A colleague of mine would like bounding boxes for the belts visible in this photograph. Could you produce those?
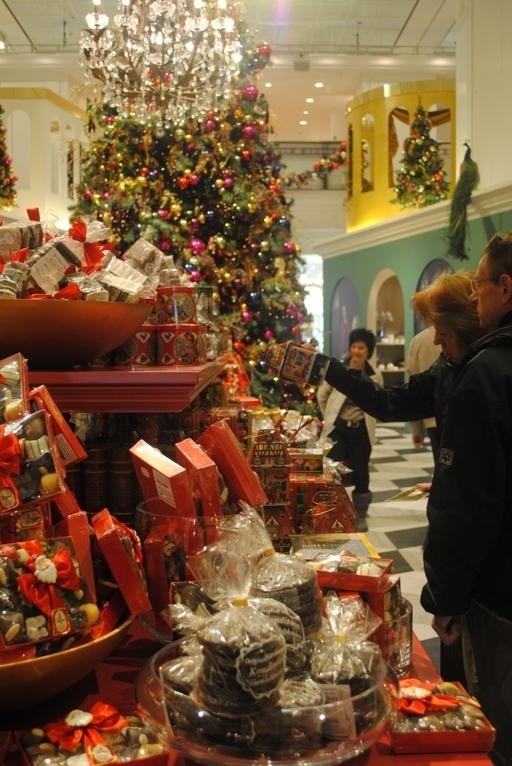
[335,416,366,430]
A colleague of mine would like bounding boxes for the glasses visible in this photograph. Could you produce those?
[469,275,499,294]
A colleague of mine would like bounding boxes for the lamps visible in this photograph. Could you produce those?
[74,0,245,135]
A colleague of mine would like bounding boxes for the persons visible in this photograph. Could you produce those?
[303,268,488,678]
[316,327,385,532]
[406,325,443,466]
[418,230,512,766]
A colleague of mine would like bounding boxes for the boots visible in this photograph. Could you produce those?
[352,488,371,532]
